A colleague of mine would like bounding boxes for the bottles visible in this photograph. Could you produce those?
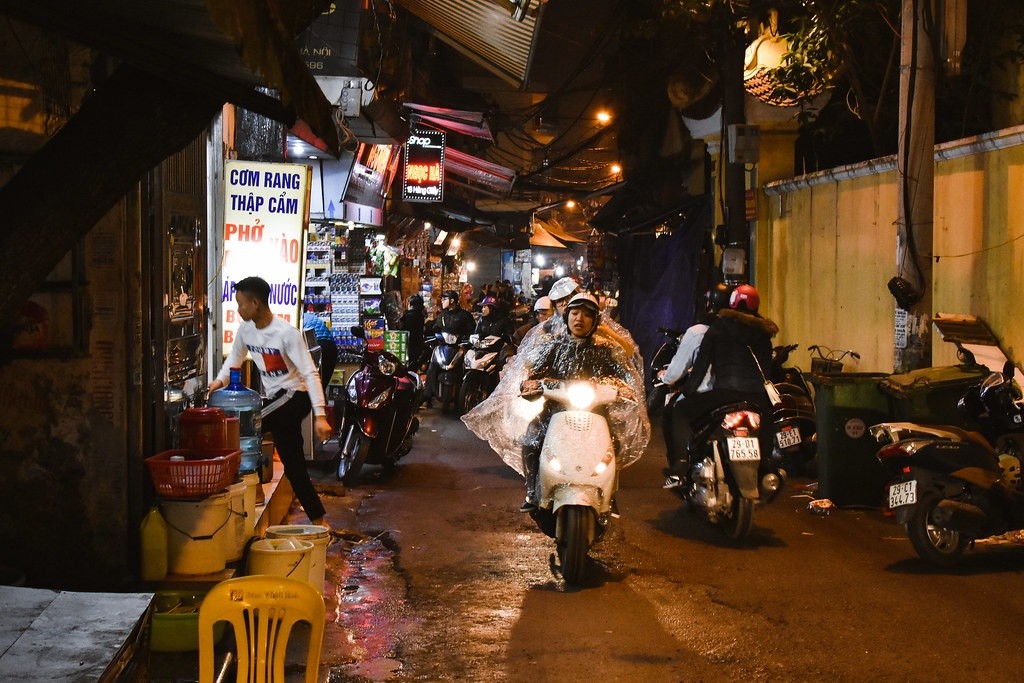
[322,244,366,273]
[140,507,168,579]
[333,327,365,363]
[208,366,261,483]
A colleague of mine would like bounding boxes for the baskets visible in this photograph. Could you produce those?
[811,358,842,375]
[145,447,243,498]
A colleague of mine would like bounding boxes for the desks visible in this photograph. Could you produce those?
[0,585,156,683]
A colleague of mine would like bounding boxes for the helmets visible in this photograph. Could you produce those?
[439,290,458,302]
[534,296,554,311]
[704,282,732,311]
[728,284,759,312]
[549,277,581,308]
[477,296,499,309]
[567,293,599,319]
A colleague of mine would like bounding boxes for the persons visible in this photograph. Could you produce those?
[398,276,558,402]
[663,285,784,491]
[499,277,644,519]
[657,282,735,477]
[205,276,334,532]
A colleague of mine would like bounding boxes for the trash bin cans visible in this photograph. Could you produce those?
[802,371,891,511]
[878,364,991,432]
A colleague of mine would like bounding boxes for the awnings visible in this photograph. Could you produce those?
[395,0,548,90]
[402,100,500,147]
[444,146,518,201]
[0,0,344,157]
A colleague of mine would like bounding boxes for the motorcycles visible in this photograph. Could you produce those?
[335,325,424,481]
[867,345,1024,564]
[422,330,517,410]
[651,326,820,543]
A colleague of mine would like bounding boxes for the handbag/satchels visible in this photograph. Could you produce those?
[764,380,782,406]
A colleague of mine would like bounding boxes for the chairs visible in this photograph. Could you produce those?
[199,574,326,683]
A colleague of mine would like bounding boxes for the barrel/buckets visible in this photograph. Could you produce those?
[226,478,248,563]
[156,489,232,575]
[265,525,331,597]
[247,539,315,621]
[239,471,260,539]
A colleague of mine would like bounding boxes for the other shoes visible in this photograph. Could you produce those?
[611,501,620,519]
[520,499,535,512]
[663,476,683,488]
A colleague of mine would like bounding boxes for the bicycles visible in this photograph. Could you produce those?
[806,344,861,379]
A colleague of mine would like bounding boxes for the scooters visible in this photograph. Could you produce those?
[517,379,633,587]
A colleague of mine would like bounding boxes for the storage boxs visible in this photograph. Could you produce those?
[381,329,408,363]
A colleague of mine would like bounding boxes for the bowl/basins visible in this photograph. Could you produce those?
[150,590,225,652]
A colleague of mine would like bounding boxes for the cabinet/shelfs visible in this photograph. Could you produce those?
[305,244,333,326]
